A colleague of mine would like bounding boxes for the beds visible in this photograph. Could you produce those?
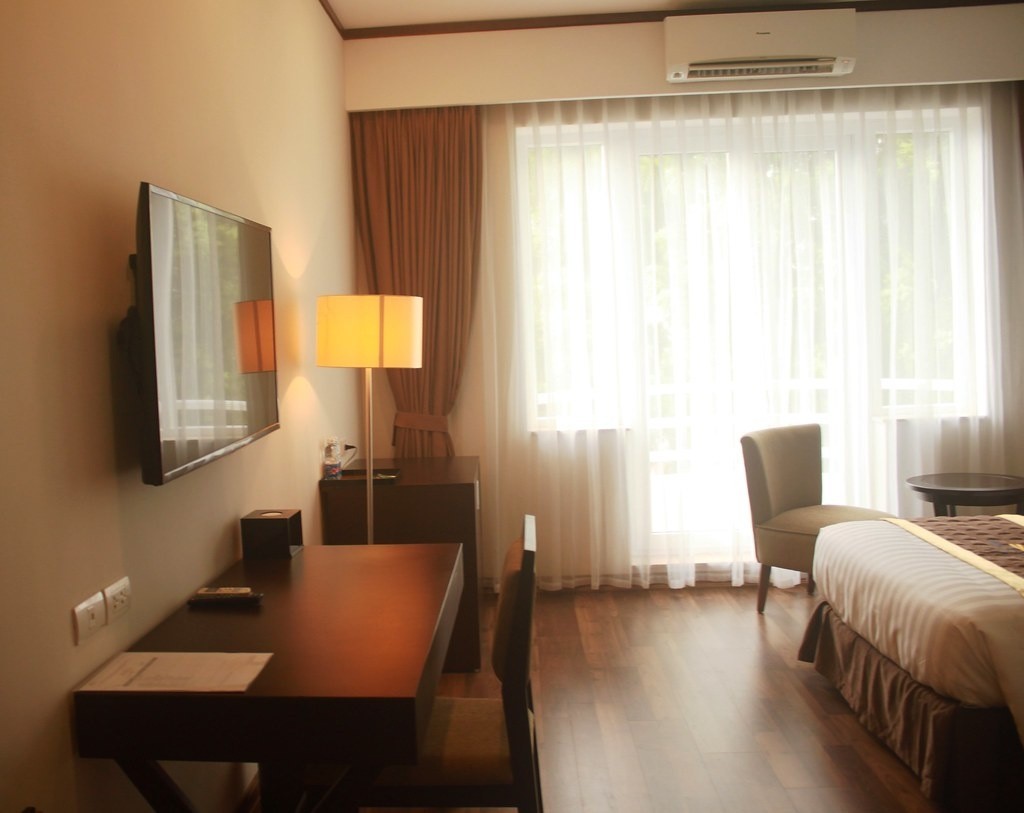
[796,514,1024,803]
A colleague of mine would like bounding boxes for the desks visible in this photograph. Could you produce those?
[72,542,464,813]
[319,455,482,674]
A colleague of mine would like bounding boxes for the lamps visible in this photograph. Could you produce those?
[314,295,422,546]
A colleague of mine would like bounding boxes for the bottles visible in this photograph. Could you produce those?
[325,436,341,480]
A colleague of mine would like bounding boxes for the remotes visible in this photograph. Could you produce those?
[186,586,264,609]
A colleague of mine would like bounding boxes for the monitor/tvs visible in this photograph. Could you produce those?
[116,181,280,487]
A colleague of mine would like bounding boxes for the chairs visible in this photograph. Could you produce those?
[333,514,544,813]
[739,423,899,613]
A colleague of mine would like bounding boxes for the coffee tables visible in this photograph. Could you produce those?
[905,472,1024,517]
